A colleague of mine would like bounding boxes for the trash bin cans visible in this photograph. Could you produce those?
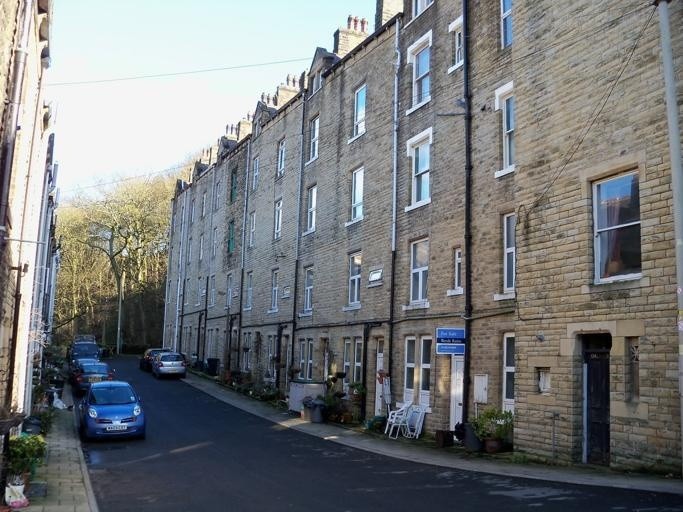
[21,417,41,435]
[464,422,481,451]
[206,358,219,375]
[312,401,324,422]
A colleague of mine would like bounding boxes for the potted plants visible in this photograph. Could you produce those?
[470,406,514,453]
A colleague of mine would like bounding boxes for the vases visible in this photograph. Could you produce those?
[8,473,28,495]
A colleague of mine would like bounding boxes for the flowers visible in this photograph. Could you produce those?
[3,432,48,472]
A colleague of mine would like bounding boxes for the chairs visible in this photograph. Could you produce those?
[382,397,429,440]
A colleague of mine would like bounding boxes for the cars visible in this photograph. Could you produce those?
[70,334,186,443]
